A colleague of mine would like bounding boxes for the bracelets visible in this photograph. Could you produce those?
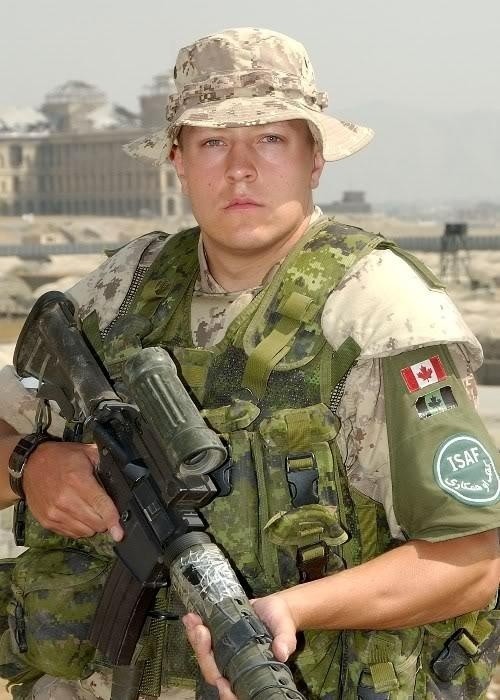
[9,429,62,500]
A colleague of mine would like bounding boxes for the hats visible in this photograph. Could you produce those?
[123,28,376,167]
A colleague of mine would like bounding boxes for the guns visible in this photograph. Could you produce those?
[13,290,306,700]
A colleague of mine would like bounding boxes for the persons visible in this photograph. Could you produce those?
[0,25,500,700]
